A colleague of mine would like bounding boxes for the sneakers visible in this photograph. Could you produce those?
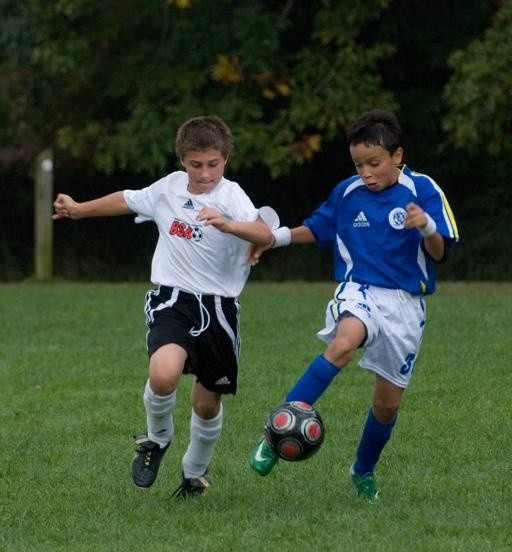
[349,460,379,503]
[178,471,216,500]
[248,434,281,476]
[129,434,171,489]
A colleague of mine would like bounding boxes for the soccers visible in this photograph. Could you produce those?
[265,401,324,462]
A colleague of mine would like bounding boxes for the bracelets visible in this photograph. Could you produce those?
[416,209,438,237]
[270,224,292,250]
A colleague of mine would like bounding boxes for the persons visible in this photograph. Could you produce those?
[51,115,281,502]
[244,107,461,500]
